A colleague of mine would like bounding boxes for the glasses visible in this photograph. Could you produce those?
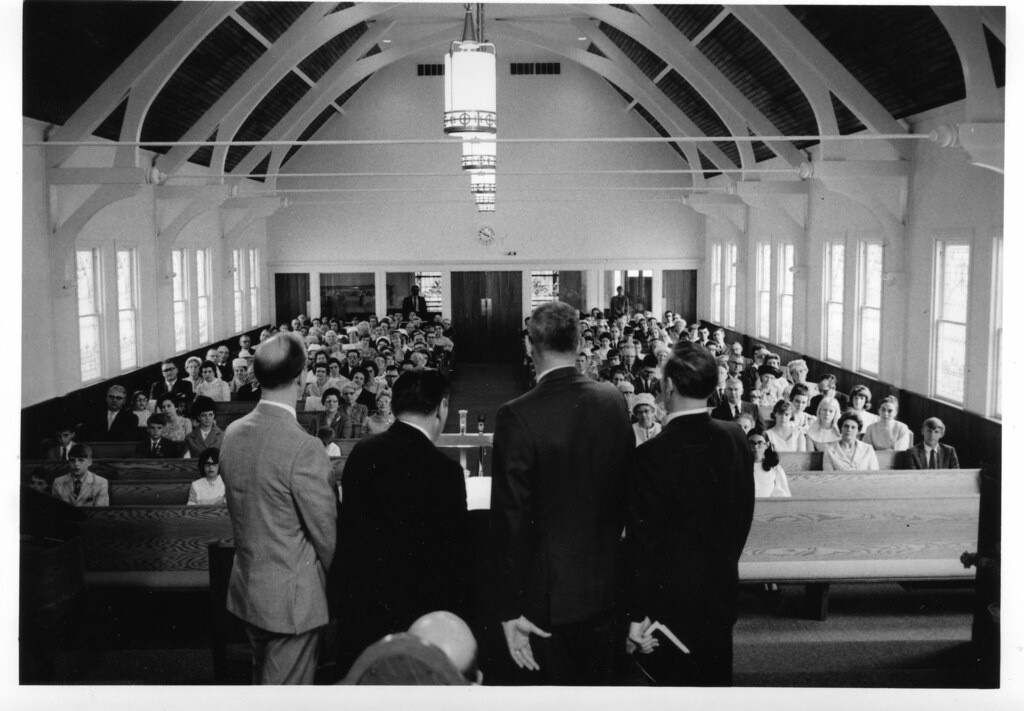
[204,462,218,467]
[751,441,766,448]
[325,399,339,404]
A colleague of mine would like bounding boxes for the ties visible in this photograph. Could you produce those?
[614,342,616,349]
[734,406,739,415]
[928,450,935,469]
[719,346,722,355]
[413,297,416,311]
[719,388,724,401]
[645,380,649,393]
[73,480,81,499]
[62,448,67,461]
[151,442,156,452]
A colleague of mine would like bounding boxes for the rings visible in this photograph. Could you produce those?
[515,650,521,653]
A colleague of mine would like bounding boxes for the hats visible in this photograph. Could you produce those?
[374,336,392,345]
[630,393,657,417]
[398,329,409,339]
[346,327,358,333]
[757,365,783,379]
[617,381,634,393]
[232,358,248,367]
[307,344,322,352]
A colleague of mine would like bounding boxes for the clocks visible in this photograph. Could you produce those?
[477,225,496,245]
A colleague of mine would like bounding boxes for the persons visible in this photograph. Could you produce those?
[220,303,755,686]
[521,286,960,497]
[29,284,455,507]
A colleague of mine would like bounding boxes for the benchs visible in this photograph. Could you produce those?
[20,398,321,600]
[648,451,982,622]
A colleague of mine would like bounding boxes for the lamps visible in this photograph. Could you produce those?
[443,3,497,214]
